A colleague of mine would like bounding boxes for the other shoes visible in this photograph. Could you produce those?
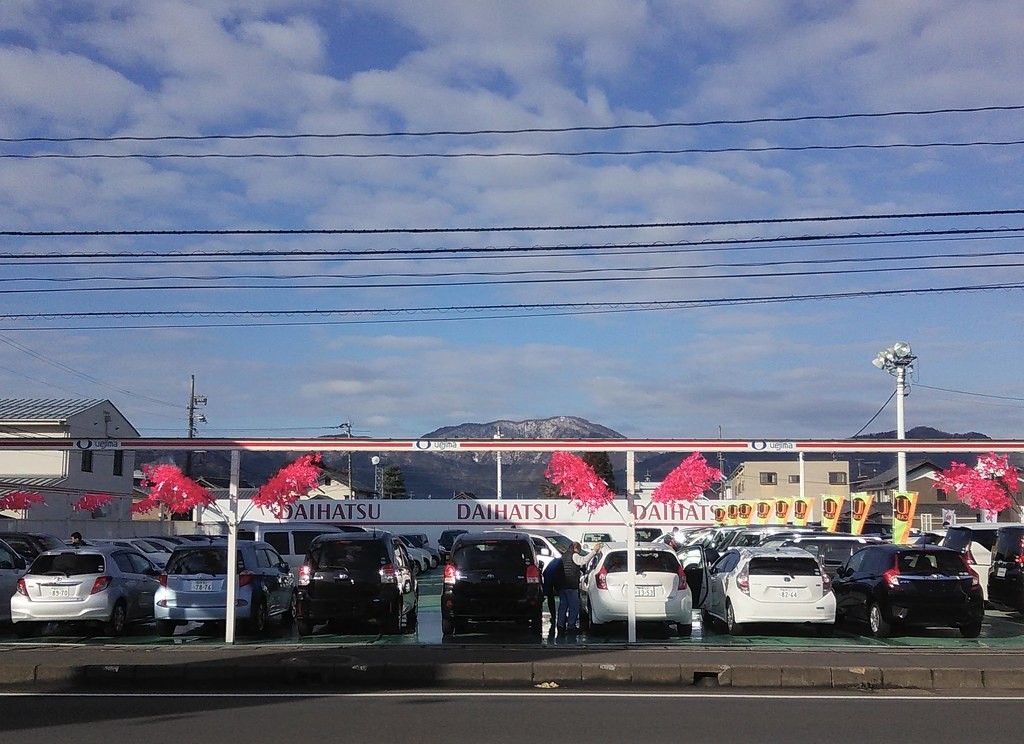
[567,627,580,630]
[558,625,566,630]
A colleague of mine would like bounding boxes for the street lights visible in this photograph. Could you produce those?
[874,341,919,502]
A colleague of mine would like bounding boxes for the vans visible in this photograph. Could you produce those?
[194,522,369,595]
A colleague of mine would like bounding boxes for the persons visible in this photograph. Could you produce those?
[942,521,949,529]
[70,532,86,546]
[664,527,682,552]
[543,541,600,635]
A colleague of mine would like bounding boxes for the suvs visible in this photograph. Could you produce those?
[438,532,550,640]
[295,530,421,634]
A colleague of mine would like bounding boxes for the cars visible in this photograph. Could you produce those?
[10,545,164,636]
[0,531,446,619]
[496,520,1024,620]
[703,547,839,635]
[579,543,710,637]
[154,538,298,638]
[832,543,985,639]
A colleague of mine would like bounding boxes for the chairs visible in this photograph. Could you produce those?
[641,558,662,570]
[611,557,626,568]
[601,536,605,540]
[587,537,592,542]
[464,548,481,567]
[915,557,937,571]
